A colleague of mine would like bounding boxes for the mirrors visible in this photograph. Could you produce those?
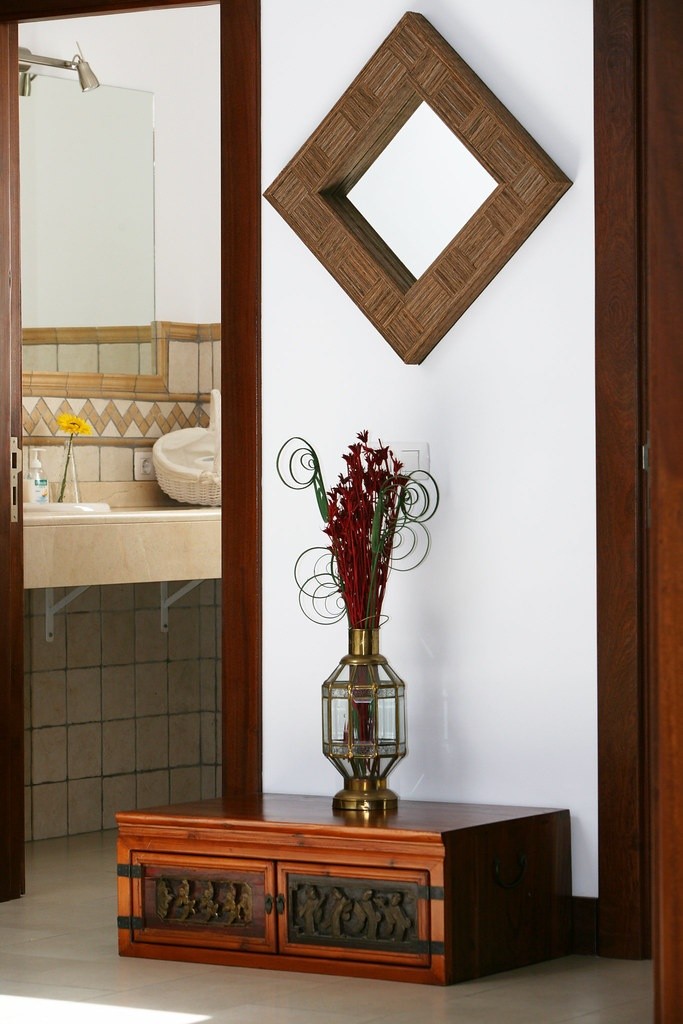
[18,76,158,378]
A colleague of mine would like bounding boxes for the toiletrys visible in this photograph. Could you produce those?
[29,447,50,503]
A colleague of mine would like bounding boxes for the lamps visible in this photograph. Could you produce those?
[18,47,100,97]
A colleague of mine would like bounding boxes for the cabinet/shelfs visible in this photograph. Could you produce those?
[115,794,571,986]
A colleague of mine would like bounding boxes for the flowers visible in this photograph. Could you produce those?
[58,411,91,503]
[276,430,439,774]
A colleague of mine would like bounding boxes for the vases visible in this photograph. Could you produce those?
[322,629,408,810]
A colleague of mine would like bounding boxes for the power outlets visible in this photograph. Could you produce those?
[133,452,157,481]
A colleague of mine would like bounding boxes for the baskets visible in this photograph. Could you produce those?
[152,388,222,507]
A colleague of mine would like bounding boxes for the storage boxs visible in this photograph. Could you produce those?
[153,427,222,506]
